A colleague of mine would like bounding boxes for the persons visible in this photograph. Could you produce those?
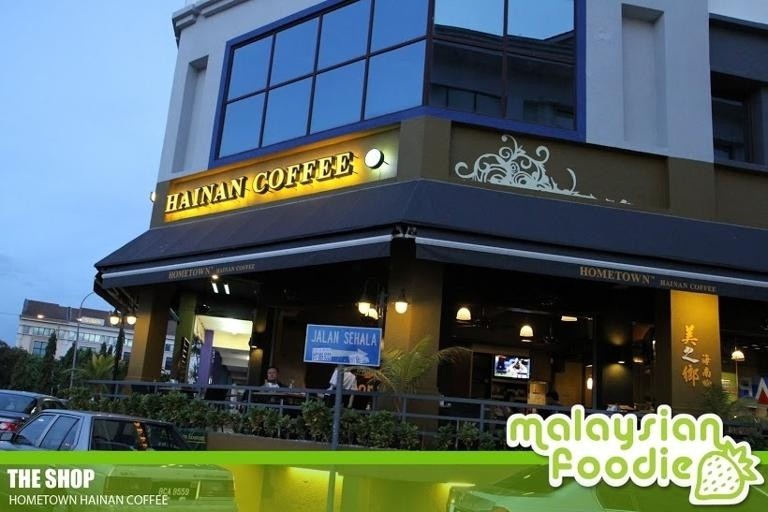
[325,367,358,409]
[504,390,518,413]
[536,390,563,420]
[267,368,296,387]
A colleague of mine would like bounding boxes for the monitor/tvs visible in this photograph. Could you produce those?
[492,353,532,382]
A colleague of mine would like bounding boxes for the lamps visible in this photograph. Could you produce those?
[586,374,594,391]
[109,296,136,325]
[355,288,409,320]
[730,346,745,396]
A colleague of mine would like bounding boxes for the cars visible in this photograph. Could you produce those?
[0,388,237,512]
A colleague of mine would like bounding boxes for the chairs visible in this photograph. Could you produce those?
[128,380,376,432]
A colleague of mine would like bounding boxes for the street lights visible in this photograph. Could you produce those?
[109,308,137,402]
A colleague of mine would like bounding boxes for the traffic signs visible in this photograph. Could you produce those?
[300,323,382,368]
